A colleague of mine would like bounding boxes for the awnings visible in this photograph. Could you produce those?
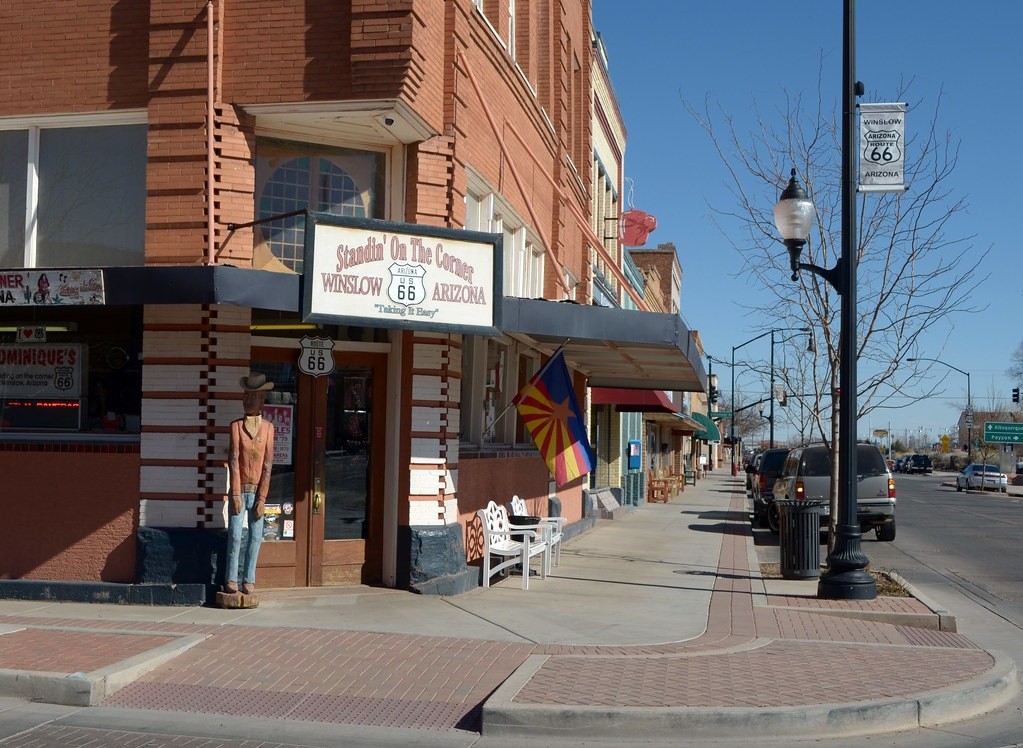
[691,412,721,441]
[592,387,678,413]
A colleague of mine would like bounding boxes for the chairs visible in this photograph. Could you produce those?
[682,463,698,487]
[479,501,550,590]
[647,465,686,504]
[506,495,567,576]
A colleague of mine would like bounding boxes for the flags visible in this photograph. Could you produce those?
[511,345,597,489]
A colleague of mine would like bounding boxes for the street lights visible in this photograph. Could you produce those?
[906,357,974,466]
[770,0,880,600]
[731,326,812,477]
[755,328,817,448]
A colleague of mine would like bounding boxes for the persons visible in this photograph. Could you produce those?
[223,371,275,593]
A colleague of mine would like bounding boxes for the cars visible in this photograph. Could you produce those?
[886,453,934,474]
[955,464,1007,493]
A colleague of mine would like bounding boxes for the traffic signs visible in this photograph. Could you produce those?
[984,421,1023,445]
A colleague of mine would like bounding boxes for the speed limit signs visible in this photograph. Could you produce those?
[855,100,909,194]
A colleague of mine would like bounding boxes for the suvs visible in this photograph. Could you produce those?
[745,448,791,529]
[766,438,898,541]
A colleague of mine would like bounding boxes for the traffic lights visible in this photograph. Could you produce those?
[710,385,719,404]
[963,444,967,450]
[1012,387,1020,403]
[835,386,840,393]
[779,390,788,406]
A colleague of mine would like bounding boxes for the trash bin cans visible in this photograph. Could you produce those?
[773,499,823,580]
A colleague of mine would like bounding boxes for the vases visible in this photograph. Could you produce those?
[507,515,540,577]
